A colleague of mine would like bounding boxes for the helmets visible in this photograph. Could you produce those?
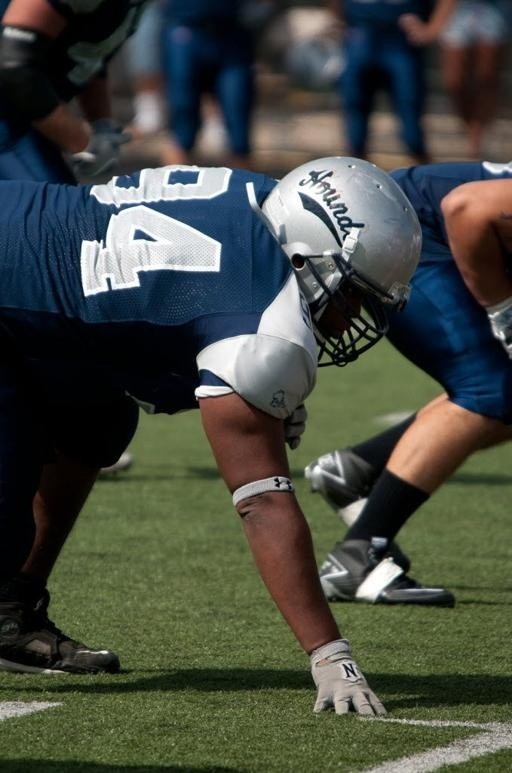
[262,156,422,320]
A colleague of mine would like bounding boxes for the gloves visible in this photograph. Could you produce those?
[283,404,306,449]
[310,639,388,714]
[74,118,130,180]
[488,304,512,362]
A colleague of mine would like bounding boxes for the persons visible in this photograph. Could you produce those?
[305,1,454,166]
[149,2,294,172]
[298,157,510,606]
[1,0,149,683]
[2,153,426,722]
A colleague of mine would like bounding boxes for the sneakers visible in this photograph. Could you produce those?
[2,595,118,676]
[303,445,411,573]
[101,452,130,477]
[318,537,455,606]
